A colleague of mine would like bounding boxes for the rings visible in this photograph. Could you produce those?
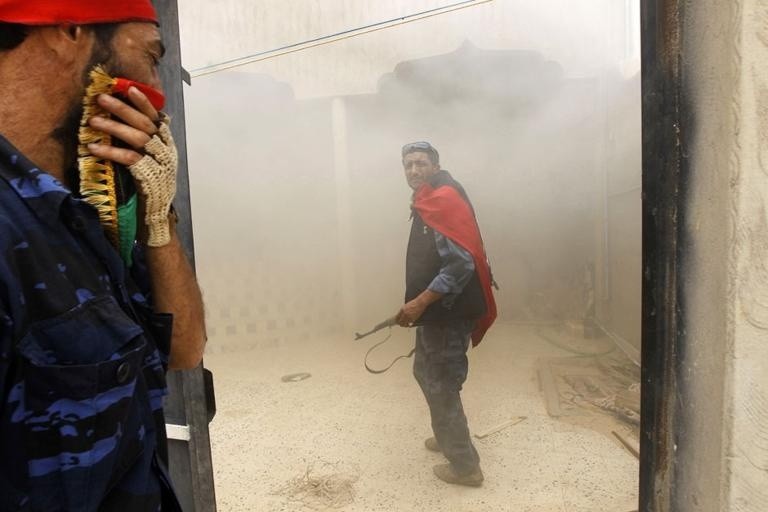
[407,322,414,326]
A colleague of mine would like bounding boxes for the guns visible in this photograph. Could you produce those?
[354,313,398,340]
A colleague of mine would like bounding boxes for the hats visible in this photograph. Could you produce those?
[1,1,160,27]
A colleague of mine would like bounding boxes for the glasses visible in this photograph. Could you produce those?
[404,141,430,151]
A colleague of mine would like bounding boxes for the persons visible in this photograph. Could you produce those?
[0,0,211,511]
[391,141,496,487]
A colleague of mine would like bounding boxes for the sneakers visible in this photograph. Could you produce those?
[426,437,440,452]
[434,464,484,486]
